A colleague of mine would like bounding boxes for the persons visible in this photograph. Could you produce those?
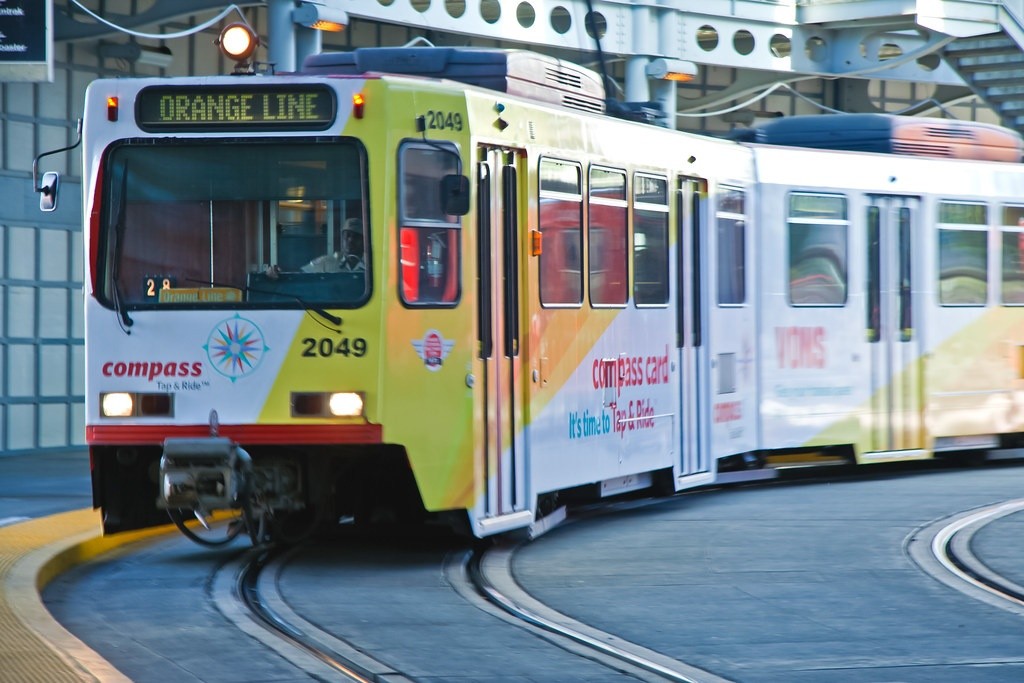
[299,218,366,271]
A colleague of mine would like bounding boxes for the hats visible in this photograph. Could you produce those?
[341,218,364,235]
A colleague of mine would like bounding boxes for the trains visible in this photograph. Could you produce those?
[30,24,1024,544]
